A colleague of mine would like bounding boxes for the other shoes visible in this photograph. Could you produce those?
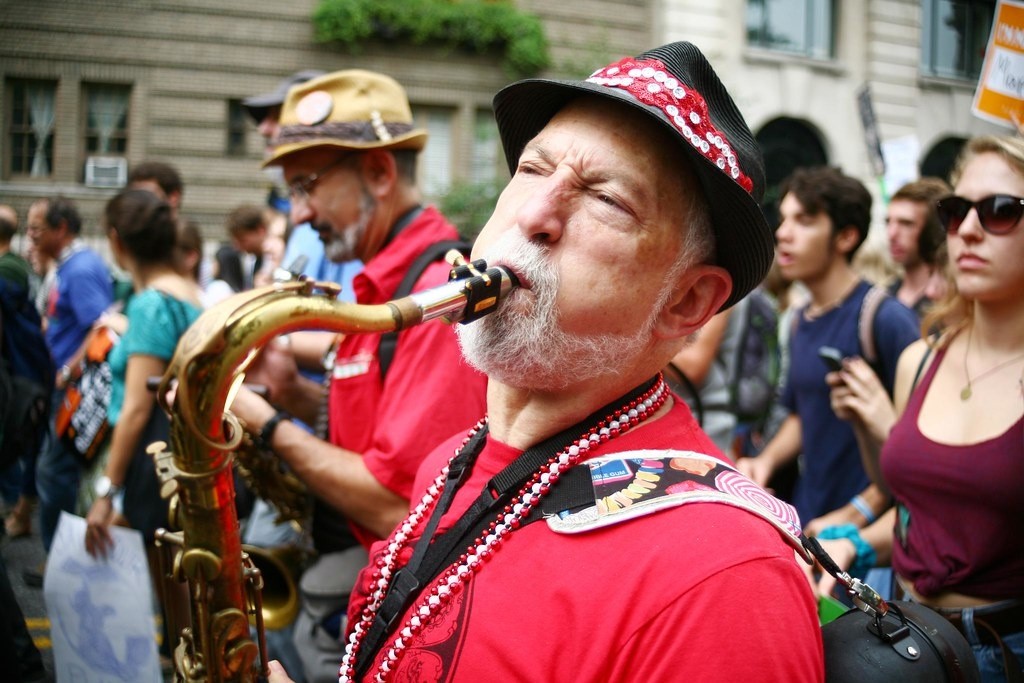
[6,499,34,537]
[22,562,46,587]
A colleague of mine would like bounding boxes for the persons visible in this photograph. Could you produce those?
[0,64,489,683]
[662,114,1024,683]
[332,41,825,683]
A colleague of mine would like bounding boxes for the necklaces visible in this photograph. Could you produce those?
[961,328,1024,399]
[337,372,669,683]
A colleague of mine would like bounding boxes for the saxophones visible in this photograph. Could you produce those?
[149,377,318,632]
[145,249,522,683]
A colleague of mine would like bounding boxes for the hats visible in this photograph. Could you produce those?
[260,68,426,172]
[494,42,777,304]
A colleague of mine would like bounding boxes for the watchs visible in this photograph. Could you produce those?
[61,365,73,382]
[93,475,118,499]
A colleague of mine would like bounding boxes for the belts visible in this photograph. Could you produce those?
[947,603,1024,683]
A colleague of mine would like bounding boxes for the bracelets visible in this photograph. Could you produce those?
[281,335,290,348]
[850,496,876,523]
[258,410,289,443]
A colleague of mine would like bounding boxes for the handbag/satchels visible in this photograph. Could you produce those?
[823,602,983,683]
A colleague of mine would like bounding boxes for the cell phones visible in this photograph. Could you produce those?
[817,346,846,387]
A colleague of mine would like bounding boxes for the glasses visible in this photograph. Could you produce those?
[937,193,1024,236]
[277,152,352,200]
